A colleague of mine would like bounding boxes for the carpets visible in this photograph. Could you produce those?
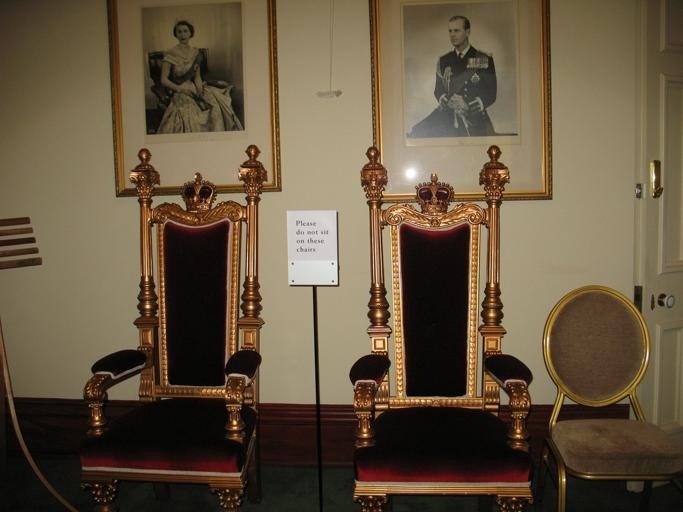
[457,52,462,61]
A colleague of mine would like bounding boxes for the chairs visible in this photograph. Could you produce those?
[80,145,268,509]
[350,144,536,508]
[534,286,683,510]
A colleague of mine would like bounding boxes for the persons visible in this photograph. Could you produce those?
[410,13,498,137]
[160,17,226,133]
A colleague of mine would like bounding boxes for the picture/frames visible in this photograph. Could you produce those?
[108,0,282,197]
[369,2,553,201]
